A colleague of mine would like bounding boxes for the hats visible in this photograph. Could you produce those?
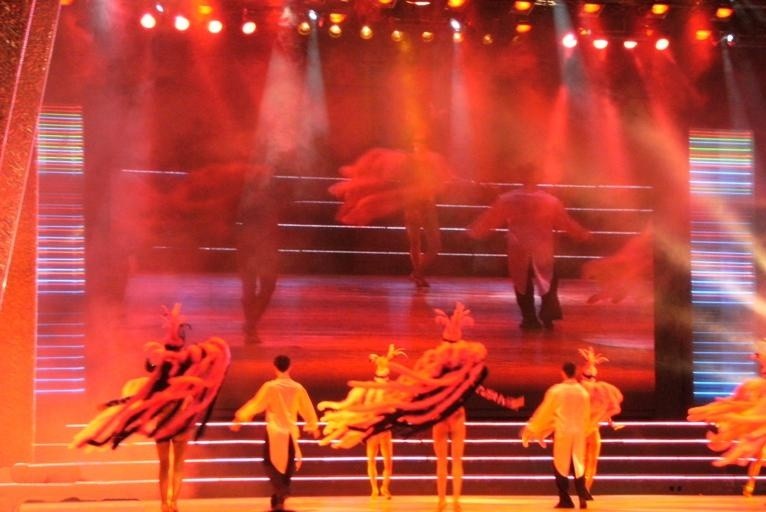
[164,336,183,346]
[583,365,596,377]
[374,368,390,376]
[442,326,462,340]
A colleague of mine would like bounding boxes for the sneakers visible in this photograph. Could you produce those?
[242,323,261,344]
[161,501,179,512]
[742,486,754,498]
[519,317,554,329]
[369,489,391,499]
[579,488,594,509]
[408,272,431,289]
[554,498,575,508]
[271,502,285,510]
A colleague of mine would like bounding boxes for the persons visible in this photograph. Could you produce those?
[685,342,765,497]
[390,127,449,285]
[225,355,320,510]
[219,152,292,352]
[575,341,625,495]
[516,361,590,510]
[134,298,206,510]
[462,158,595,330]
[316,345,398,504]
[412,300,526,512]
